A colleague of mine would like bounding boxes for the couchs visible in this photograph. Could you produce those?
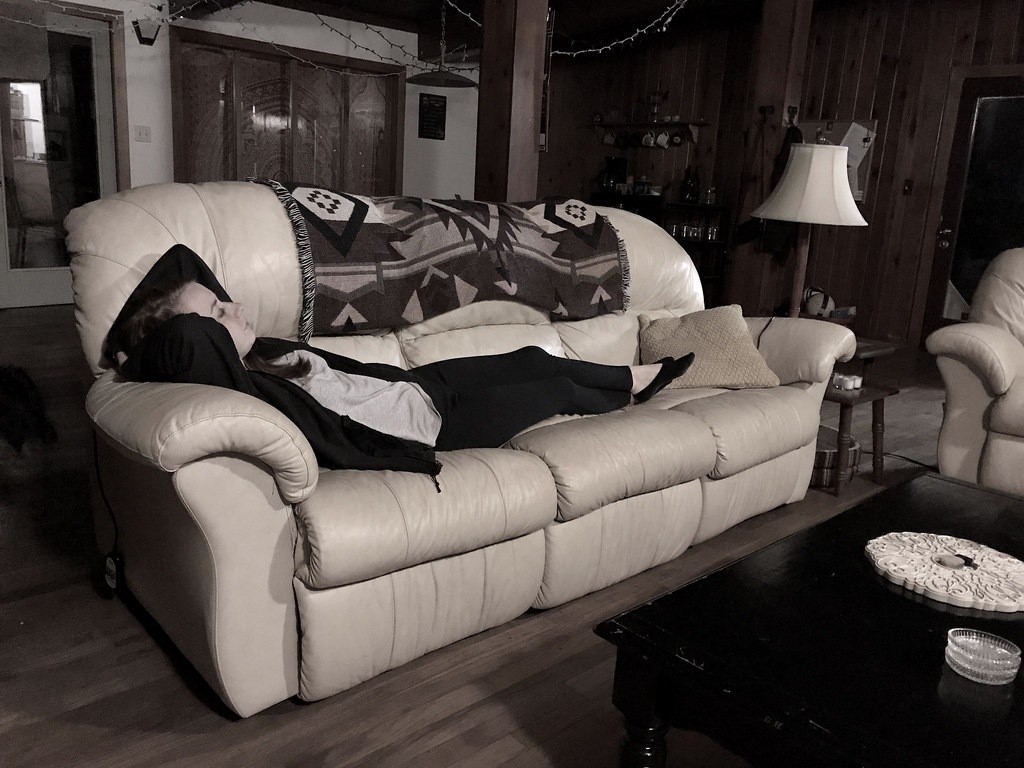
[926,248,1024,496]
[63,184,857,719]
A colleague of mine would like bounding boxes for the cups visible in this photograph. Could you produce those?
[655,130,671,149]
[668,224,719,240]
[603,129,617,145]
[642,129,656,146]
[649,185,663,195]
[663,115,680,121]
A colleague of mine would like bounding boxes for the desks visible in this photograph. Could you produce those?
[593,470,1024,768]
[824,338,900,496]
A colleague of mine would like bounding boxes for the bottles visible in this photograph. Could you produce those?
[649,102,659,122]
[624,169,633,195]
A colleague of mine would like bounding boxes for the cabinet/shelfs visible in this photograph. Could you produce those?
[590,192,731,309]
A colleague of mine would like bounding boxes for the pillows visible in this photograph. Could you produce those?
[638,305,781,389]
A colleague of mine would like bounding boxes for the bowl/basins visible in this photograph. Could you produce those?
[808,425,863,488]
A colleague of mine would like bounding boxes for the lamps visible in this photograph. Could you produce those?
[749,143,868,318]
[405,0,477,88]
